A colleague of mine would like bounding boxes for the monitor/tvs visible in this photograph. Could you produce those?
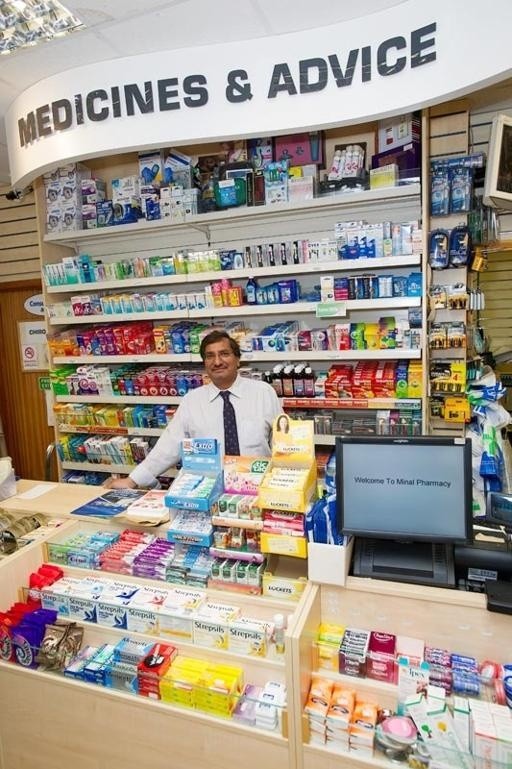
[482,110,512,212]
[335,436,475,547]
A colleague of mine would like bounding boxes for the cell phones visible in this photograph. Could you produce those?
[467,567,498,582]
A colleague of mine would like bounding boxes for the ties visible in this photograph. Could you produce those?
[219,390,239,456]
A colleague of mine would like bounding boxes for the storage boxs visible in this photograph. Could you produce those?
[40,149,199,235]
[307,537,355,589]
[376,113,419,157]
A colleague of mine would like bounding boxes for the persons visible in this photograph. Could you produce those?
[102,330,289,490]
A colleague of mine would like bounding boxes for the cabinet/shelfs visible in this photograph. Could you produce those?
[1,512,511,767]
[26,112,429,489]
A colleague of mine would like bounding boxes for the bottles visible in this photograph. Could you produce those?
[264,364,316,398]
